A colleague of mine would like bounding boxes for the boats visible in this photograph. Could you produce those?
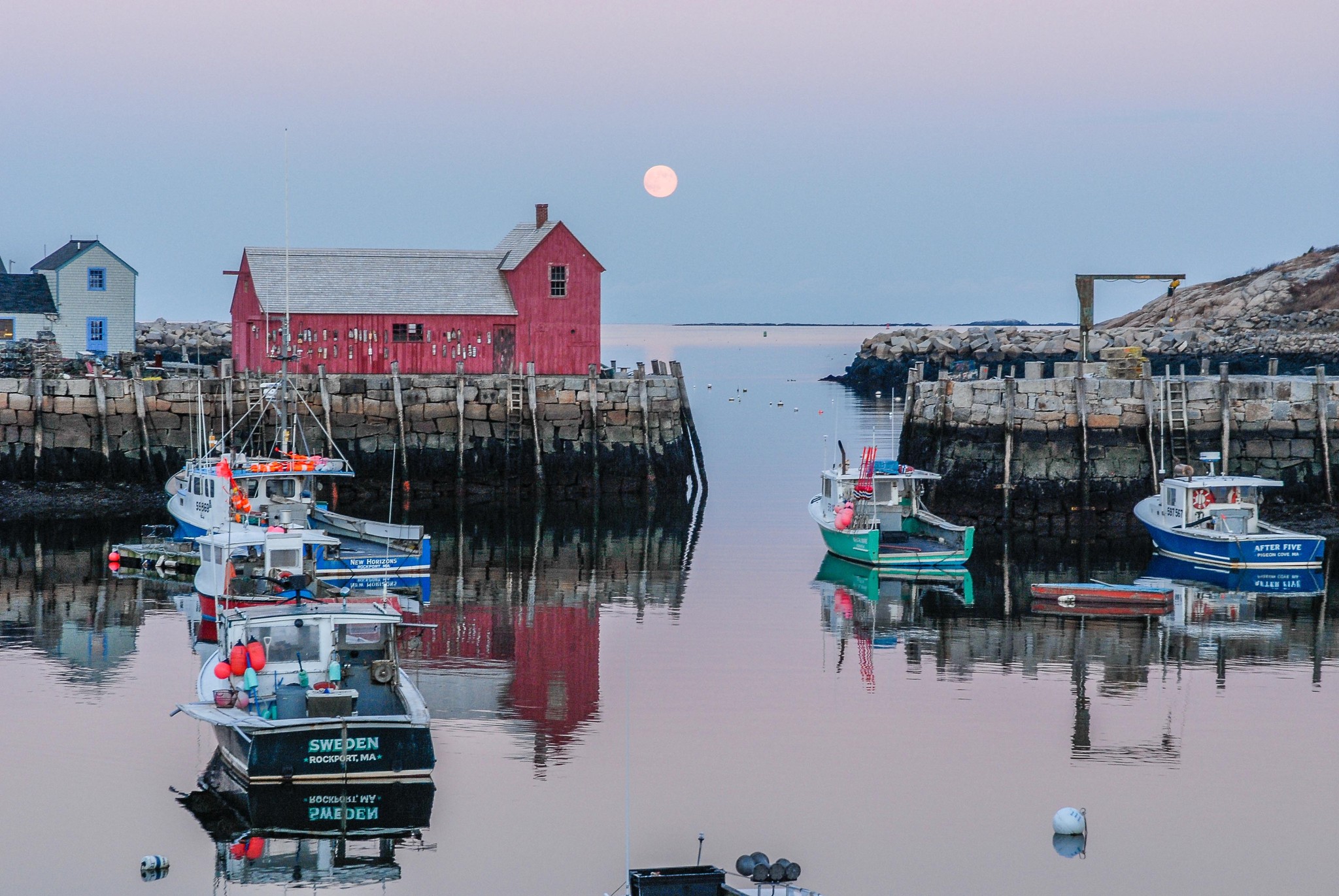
[104,215,436,784]
[1132,449,1328,569]
[1030,582,1175,605]
[806,385,976,566]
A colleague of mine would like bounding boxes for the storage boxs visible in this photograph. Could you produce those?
[882,530,909,542]
[855,519,881,531]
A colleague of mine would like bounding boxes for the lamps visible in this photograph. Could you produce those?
[340,587,350,609]
[735,853,801,883]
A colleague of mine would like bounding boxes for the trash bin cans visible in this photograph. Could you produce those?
[221,359,234,378]
[275,687,307,719]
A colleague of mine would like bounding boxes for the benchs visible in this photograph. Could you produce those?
[1054,362,1104,378]
[1024,362,1044,380]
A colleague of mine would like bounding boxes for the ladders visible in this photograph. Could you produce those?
[244,366,267,458]
[1165,364,1191,478]
[505,360,523,492]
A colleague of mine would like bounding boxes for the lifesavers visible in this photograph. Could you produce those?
[275,571,294,592]
[310,682,339,690]
[1193,489,1210,509]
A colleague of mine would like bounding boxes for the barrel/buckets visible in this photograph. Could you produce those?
[275,686,307,720]
[279,510,292,528]
[230,578,254,596]
